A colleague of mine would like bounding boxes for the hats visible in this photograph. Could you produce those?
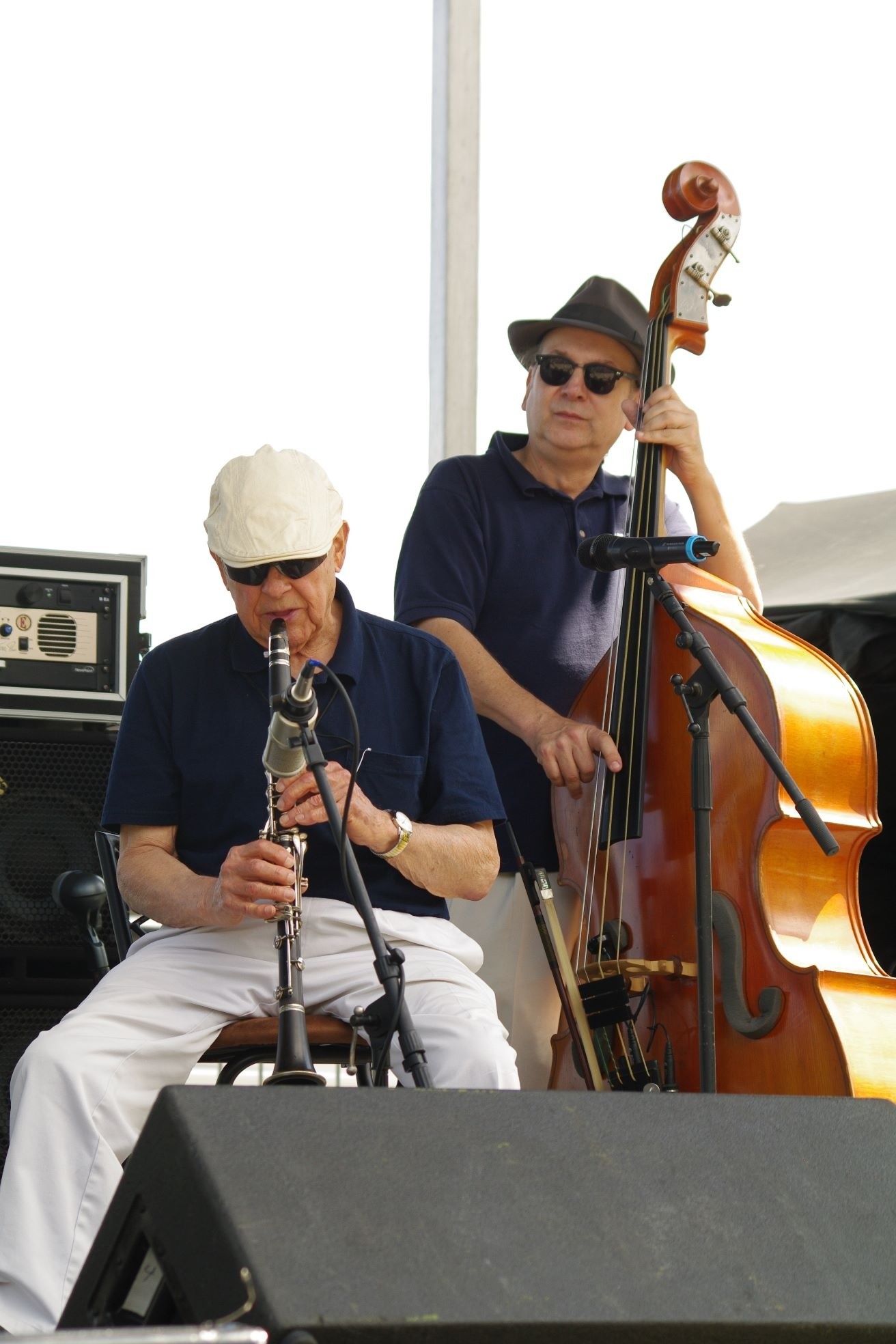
[507,275,675,395]
[203,443,344,569]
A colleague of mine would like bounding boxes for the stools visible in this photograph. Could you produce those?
[198,1015,381,1089]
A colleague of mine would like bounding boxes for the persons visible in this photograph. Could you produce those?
[0,445,521,1336]
[392,278,765,1089]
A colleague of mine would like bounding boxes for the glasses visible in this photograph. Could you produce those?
[534,353,642,394]
[225,554,327,586]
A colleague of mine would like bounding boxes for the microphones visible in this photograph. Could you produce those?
[51,867,107,914]
[577,532,721,575]
[261,657,317,778]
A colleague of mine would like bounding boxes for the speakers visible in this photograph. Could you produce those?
[48,1083,896,1343]
[0,724,131,1175]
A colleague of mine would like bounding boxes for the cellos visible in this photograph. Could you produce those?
[541,161,894,1102]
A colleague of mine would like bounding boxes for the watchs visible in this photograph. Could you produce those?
[370,809,413,859]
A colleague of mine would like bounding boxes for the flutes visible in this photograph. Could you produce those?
[259,620,331,1087]
